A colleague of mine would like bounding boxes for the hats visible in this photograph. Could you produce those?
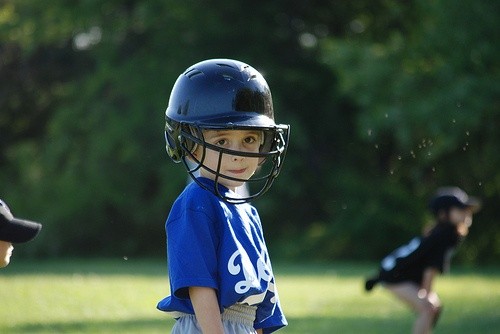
[0,199,42,243]
[427,188,479,210]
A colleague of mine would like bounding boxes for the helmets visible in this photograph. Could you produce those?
[164,59,288,163]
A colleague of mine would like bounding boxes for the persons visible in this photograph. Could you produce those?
[156,58,288,334]
[0,201,42,268]
[364,188,480,334]
